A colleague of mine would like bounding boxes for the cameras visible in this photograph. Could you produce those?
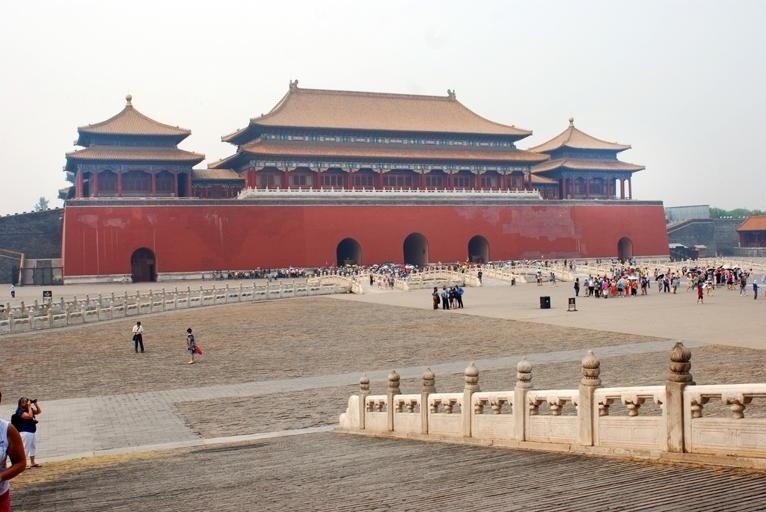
[27,397,37,404]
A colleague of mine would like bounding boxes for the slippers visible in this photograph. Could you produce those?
[31,464,42,467]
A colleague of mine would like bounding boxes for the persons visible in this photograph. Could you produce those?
[654,265,758,304]
[510,260,557,287]
[15,396,42,469]
[0,389,26,512]
[564,258,651,299]
[432,257,483,310]
[131,321,145,353]
[186,328,197,364]
[10,283,15,297]
[211,256,431,291]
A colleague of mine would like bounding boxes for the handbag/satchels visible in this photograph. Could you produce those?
[11,414,20,432]
[133,335,136,340]
